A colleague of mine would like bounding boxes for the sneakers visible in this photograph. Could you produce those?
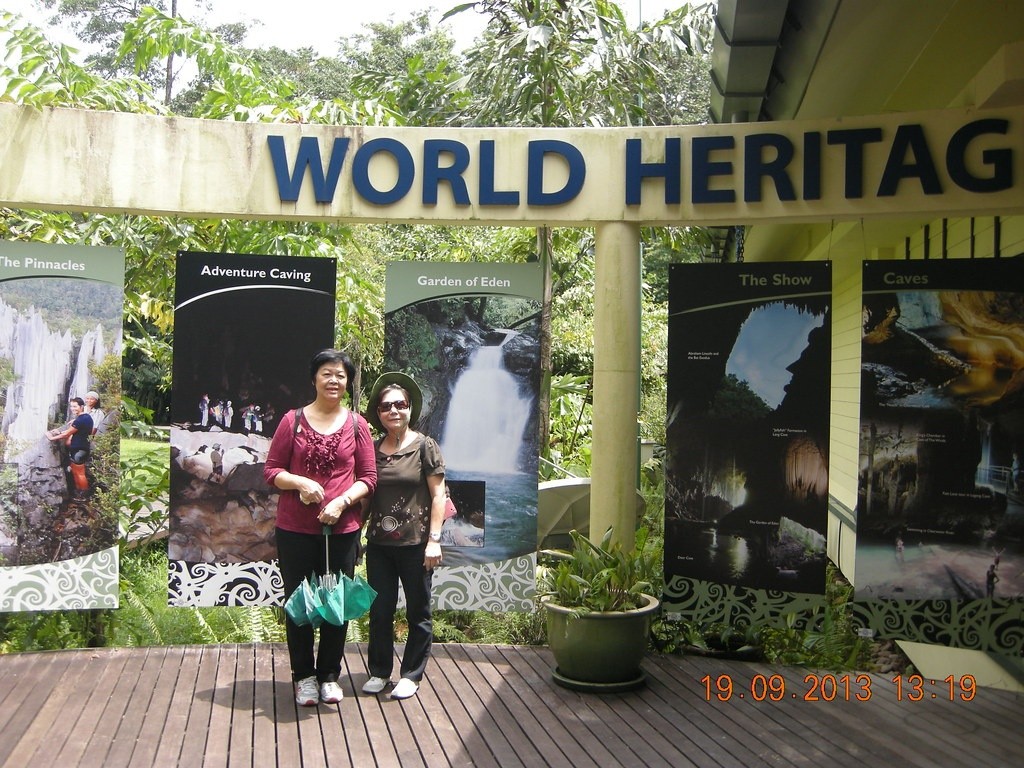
[391,678,420,698]
[362,676,390,693]
[294,675,319,705]
[320,682,344,702]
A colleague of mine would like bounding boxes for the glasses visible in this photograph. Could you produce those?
[378,400,409,412]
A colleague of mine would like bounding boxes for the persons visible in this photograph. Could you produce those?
[1011,452,1022,494]
[264,351,378,708]
[986,563,1000,599]
[896,537,903,551]
[45,391,107,502]
[360,371,447,700]
[200,392,263,484]
[992,546,1005,569]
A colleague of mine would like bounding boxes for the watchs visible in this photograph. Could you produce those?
[341,493,353,507]
[429,532,441,541]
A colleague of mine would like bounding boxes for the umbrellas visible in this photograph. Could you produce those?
[284,524,378,629]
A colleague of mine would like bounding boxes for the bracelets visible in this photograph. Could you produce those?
[60,432,61,434]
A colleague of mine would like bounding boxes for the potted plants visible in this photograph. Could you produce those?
[535,529,661,692]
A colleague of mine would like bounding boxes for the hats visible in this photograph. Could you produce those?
[366,372,423,432]
